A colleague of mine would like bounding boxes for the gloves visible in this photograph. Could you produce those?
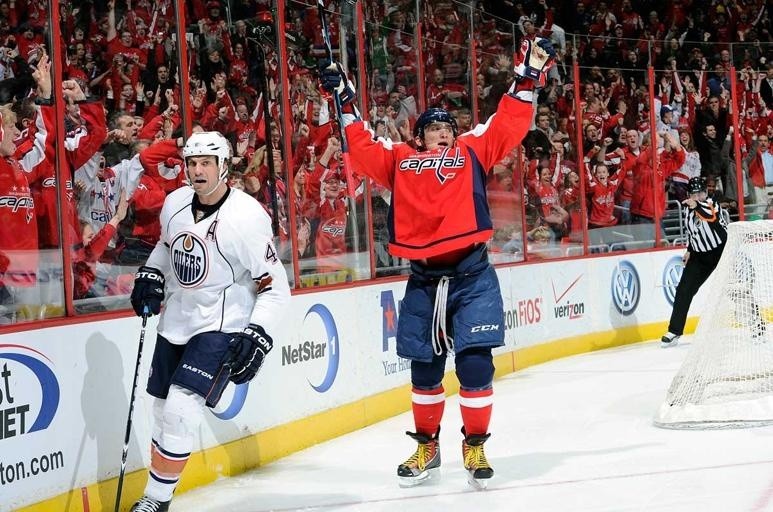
[318,61,357,106]
[225,323,274,386]
[509,32,559,90]
[128,264,167,317]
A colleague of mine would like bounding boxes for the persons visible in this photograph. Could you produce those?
[317,36,557,480]
[0,0,772,344]
[129,132,291,511]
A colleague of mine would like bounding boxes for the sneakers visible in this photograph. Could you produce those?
[125,494,173,512]
[660,330,682,344]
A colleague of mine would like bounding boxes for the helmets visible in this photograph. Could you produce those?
[412,105,459,138]
[684,176,708,194]
[179,129,232,172]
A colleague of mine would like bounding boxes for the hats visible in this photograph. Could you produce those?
[660,104,678,114]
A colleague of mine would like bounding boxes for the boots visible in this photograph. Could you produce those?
[458,425,495,479]
[394,423,442,478]
